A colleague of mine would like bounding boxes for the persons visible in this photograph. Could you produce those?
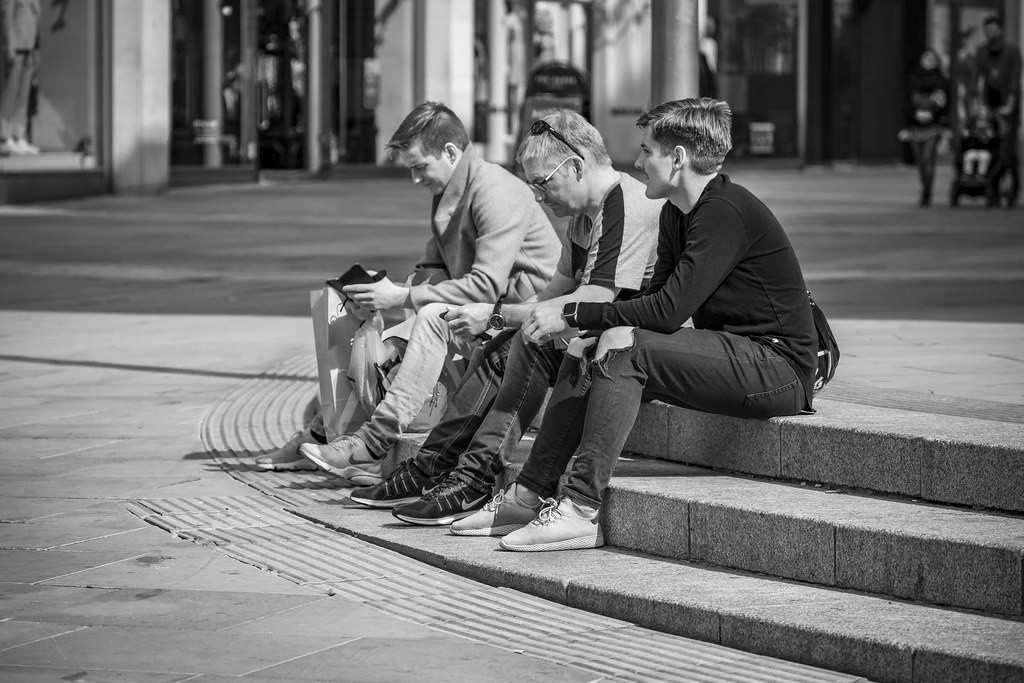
[963,15,1024,207]
[351,106,667,524]
[0,0,40,155]
[256,100,563,472]
[898,46,954,207]
[450,98,819,551]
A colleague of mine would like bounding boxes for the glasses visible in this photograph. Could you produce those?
[530,120,586,161]
[527,157,574,192]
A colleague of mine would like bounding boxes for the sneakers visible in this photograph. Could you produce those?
[450,488,550,535]
[391,477,492,526]
[499,494,604,551]
[350,457,444,510]
[255,428,324,470]
[299,435,383,485]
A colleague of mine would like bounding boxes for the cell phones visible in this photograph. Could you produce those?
[325,263,376,304]
[439,310,492,343]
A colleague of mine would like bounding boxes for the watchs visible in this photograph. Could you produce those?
[563,302,579,329]
[489,302,505,330]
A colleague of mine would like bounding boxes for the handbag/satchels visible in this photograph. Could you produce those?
[811,304,840,396]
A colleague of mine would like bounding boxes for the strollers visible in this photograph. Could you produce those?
[950,110,1008,209]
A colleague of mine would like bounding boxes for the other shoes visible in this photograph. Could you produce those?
[16,139,40,155]
[0,138,20,154]
